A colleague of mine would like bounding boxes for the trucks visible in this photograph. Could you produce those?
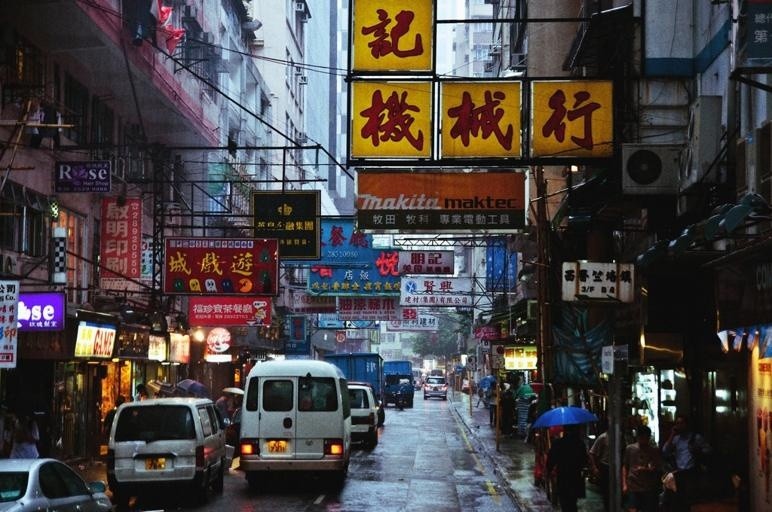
[383,359,413,376]
[322,349,385,405]
[384,373,416,408]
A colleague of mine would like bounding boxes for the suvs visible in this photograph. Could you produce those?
[347,386,380,452]
[421,375,449,400]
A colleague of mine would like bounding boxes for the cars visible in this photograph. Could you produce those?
[0,456,116,512]
[412,368,446,390]
[348,382,384,426]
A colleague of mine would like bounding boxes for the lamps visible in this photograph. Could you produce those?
[636,192,772,269]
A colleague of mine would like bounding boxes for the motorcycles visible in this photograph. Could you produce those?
[391,386,407,411]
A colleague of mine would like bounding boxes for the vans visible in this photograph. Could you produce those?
[237,353,354,484]
[102,395,229,498]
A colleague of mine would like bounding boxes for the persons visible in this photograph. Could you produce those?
[2,369,245,476]
[455,370,731,511]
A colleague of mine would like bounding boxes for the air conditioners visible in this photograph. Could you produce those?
[300,132,307,143]
[527,298,539,320]
[204,32,214,44]
[301,75,308,84]
[186,5,199,18]
[492,345,504,355]
[1,253,16,274]
[296,3,305,12]
[512,54,525,65]
[620,142,681,193]
[301,14,308,22]
[489,46,501,56]
